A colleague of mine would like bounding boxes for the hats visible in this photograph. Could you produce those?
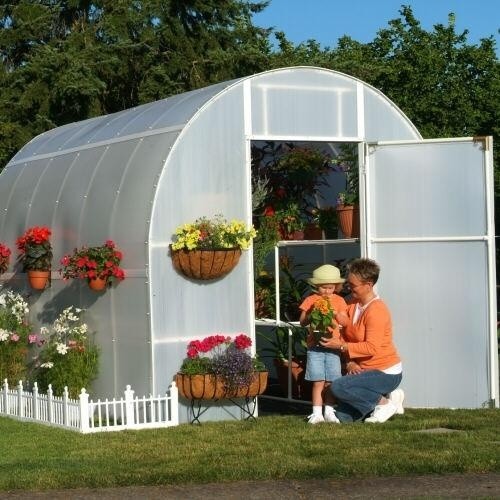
[307,264,346,289]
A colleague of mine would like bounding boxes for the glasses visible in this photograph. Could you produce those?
[349,283,369,289]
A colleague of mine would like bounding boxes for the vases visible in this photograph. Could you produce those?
[174,366,271,403]
[28,271,51,291]
[87,271,110,293]
[168,245,243,282]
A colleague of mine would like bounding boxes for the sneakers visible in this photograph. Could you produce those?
[365,399,398,423]
[390,388,405,414]
[325,410,340,424]
[307,413,325,424]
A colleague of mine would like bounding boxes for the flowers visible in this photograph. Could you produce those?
[168,214,259,258]
[176,333,265,394]
[250,140,361,409]
[55,240,126,285]
[0,243,13,272]
[13,223,56,273]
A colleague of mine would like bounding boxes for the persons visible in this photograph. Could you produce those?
[320,255,405,423]
[299,263,349,424]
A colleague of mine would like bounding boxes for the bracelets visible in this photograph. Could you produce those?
[338,341,346,354]
